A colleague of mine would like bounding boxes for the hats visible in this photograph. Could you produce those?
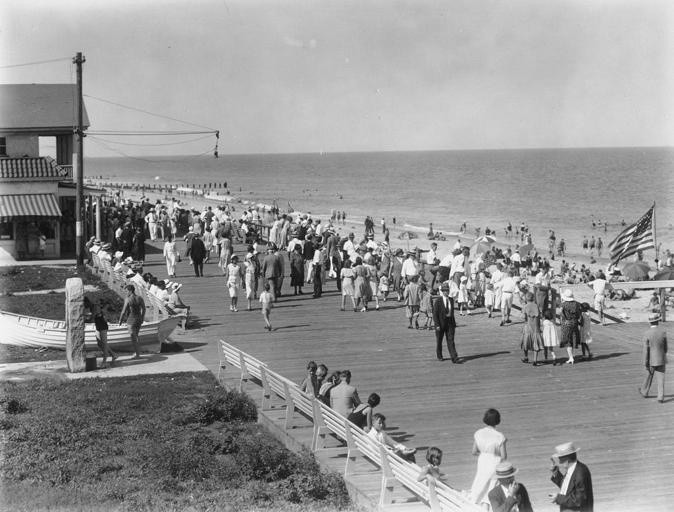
[192,231,200,238]
[171,283,183,293]
[90,232,144,294]
[190,205,226,227]
[228,245,280,263]
[492,462,519,480]
[163,278,173,289]
[407,247,418,256]
[289,226,390,253]
[560,289,575,303]
[645,312,663,325]
[148,206,168,215]
[551,442,582,461]
[438,281,453,292]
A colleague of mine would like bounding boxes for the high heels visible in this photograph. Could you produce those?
[520,357,530,364]
[566,358,575,366]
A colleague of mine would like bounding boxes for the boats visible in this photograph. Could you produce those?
[1,309,188,354]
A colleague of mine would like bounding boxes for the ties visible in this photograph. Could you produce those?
[444,299,450,315]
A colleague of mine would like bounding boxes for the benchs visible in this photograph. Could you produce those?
[83,242,191,329]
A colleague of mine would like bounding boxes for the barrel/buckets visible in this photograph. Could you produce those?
[84,355,98,371]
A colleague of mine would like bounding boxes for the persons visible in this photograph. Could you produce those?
[81,176,674,511]
[15,219,48,261]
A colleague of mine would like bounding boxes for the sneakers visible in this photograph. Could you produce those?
[657,398,665,404]
[533,355,558,366]
[580,350,595,361]
[167,255,214,278]
[638,387,649,398]
[457,310,516,327]
[277,291,325,300]
[338,304,381,315]
[381,297,404,303]
[452,357,466,364]
[263,326,278,332]
[400,446,417,460]
[228,306,257,315]
[407,322,434,332]
[436,354,445,363]
